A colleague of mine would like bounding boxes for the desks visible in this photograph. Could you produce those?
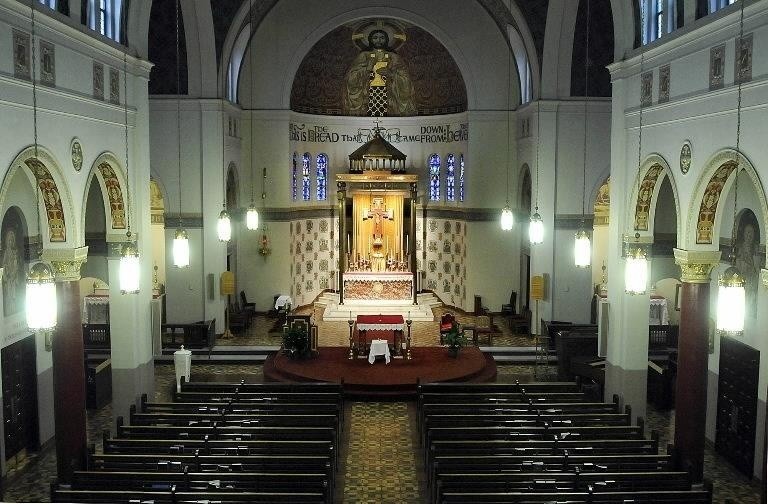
[371,340,388,366]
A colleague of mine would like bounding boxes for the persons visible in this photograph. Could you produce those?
[344,30,416,116]
[2,231,18,315]
[736,224,759,274]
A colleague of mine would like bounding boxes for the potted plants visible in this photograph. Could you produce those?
[441,328,468,358]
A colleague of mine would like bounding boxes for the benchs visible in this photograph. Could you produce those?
[412,368,715,503]
[43,372,348,502]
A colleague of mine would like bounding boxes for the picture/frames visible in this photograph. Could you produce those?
[673,284,683,311]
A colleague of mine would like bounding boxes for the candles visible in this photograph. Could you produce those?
[350,310,352,321]
[346,233,410,265]
[314,300,315,309]
[286,300,288,309]
[408,311,410,322]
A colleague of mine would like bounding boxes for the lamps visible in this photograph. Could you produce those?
[497,1,597,272]
[529,275,545,345]
[115,1,146,297]
[21,0,60,334]
[716,0,749,340]
[214,1,260,245]
[171,0,193,270]
[625,0,652,299]
[219,272,238,342]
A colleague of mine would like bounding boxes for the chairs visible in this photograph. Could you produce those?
[473,310,493,346]
[223,289,256,335]
[502,289,532,337]
[440,313,461,341]
